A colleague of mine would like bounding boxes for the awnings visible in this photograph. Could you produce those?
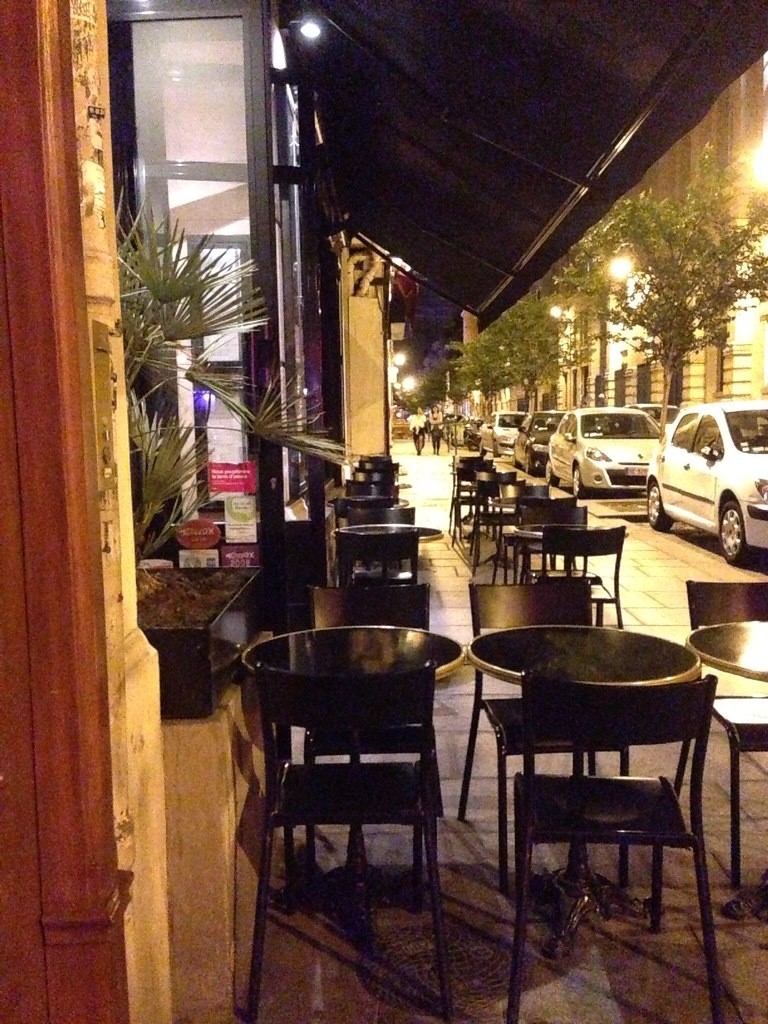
[260,1,768,332]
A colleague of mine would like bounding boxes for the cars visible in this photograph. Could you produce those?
[512,410,565,472]
[622,402,681,439]
[480,410,530,457]
[646,400,768,564]
[544,406,663,498]
[463,417,484,451]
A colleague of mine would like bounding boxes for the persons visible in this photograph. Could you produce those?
[410,406,427,456]
[429,405,442,455]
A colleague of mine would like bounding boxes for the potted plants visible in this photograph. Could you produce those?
[100,186,356,718]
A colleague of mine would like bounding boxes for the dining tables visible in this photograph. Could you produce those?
[330,523,444,586]
[466,625,702,958]
[350,470,407,476]
[344,482,412,490]
[684,621,768,919]
[240,626,465,925]
[325,496,409,510]
[514,524,629,583]
[489,497,554,585]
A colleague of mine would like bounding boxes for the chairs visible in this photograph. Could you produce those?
[333,452,418,586]
[448,455,627,630]
[674,580,768,887]
[456,577,630,894]
[245,660,454,1024]
[506,670,723,1024]
[303,583,445,913]
